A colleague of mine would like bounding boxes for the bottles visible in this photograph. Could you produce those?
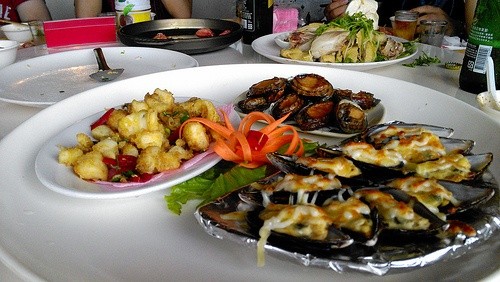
[460,0,500,94]
[115,0,153,31]
[243,0,272,45]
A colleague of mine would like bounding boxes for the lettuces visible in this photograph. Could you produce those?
[164,142,322,215]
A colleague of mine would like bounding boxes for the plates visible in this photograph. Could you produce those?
[34,97,242,197]
[232,89,384,138]
[0,64,500,282]
[0,48,199,108]
[197,167,499,268]
[251,29,419,71]
[119,19,244,53]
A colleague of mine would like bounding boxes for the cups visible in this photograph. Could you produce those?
[389,10,467,70]
[99,12,156,20]
[29,20,46,46]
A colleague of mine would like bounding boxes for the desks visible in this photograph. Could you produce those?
[0,38,500,282]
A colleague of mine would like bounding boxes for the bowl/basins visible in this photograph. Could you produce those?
[43,15,117,48]
[476,91,500,117]
[0,23,38,67]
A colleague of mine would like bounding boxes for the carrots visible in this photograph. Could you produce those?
[179,108,304,179]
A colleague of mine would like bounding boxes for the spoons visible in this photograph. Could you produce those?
[91,47,123,83]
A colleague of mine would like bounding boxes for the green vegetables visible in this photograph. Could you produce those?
[316,11,441,68]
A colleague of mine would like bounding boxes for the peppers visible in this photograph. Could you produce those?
[89,109,155,184]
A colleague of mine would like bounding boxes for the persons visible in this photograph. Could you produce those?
[75,0,193,25]
[0,0,53,26]
[325,0,468,42]
